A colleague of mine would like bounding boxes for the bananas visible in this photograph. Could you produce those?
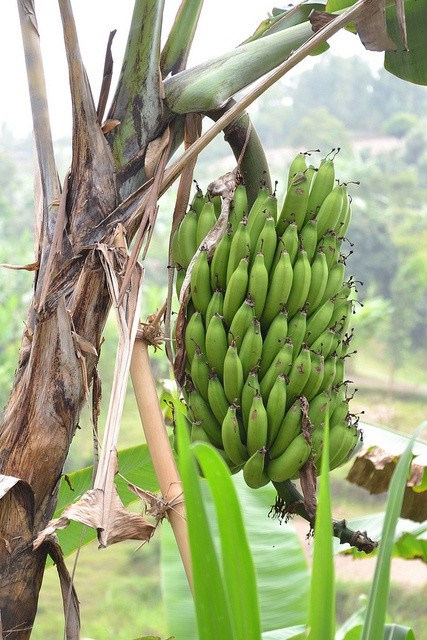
[171,150,363,490]
